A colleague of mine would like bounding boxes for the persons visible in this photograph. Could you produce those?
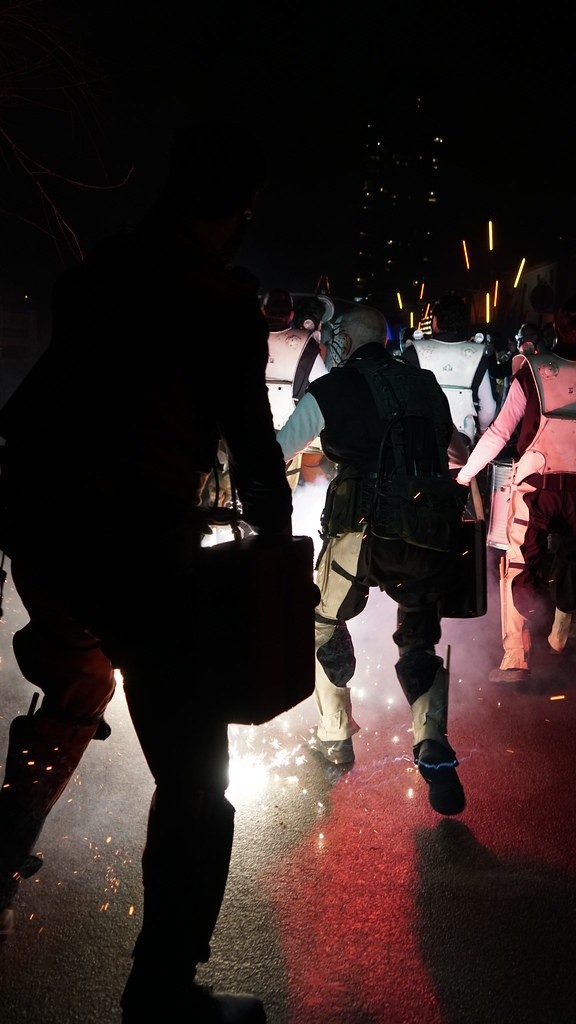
[1,121,292,1024]
[458,317,576,682]
[277,297,467,818]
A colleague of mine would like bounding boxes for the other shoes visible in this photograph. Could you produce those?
[121,984,267,1024]
[544,638,561,655]
[488,668,531,690]
[306,726,356,764]
[418,740,466,816]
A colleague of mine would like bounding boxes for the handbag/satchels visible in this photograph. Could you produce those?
[364,475,487,619]
